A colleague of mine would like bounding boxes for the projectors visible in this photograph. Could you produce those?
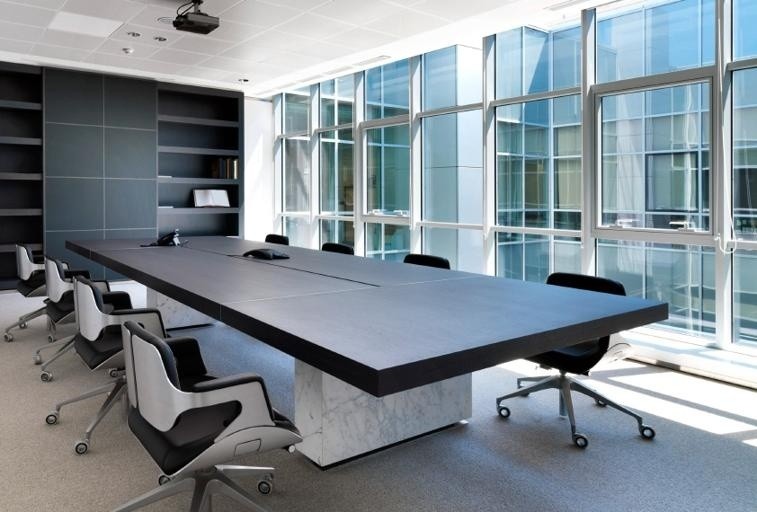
[173,12,220,35]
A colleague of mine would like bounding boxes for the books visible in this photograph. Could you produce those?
[208,156,238,180]
[192,188,231,208]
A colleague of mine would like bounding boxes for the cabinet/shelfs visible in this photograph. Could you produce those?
[159,82,245,240]
[1,61,44,290]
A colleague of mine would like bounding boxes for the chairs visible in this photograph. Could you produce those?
[496,271,657,449]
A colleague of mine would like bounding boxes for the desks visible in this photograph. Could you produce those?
[66,236,670,472]
